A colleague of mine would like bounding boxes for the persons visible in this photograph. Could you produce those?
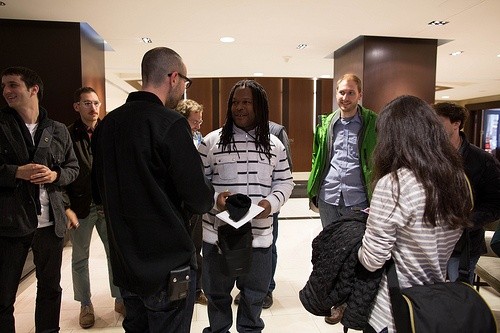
[0,67,80,333]
[234,121,293,307]
[307,74,379,325]
[175,99,209,305]
[357,94,474,333]
[63,86,127,329]
[197,80,296,333]
[91,46,215,333]
[432,102,500,286]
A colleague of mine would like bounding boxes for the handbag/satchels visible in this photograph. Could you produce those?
[391,282,498,333]
[216,222,254,280]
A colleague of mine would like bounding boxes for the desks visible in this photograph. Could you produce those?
[474,256,500,294]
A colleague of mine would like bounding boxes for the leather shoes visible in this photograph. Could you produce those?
[324,303,347,324]
[234,290,242,304]
[262,291,273,308]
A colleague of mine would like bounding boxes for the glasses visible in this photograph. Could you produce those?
[187,118,203,125]
[77,101,101,107]
[167,72,192,89]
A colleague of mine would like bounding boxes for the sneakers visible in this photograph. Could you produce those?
[115,299,126,314]
[79,303,95,327]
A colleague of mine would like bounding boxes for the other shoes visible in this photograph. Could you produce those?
[194,292,208,304]
